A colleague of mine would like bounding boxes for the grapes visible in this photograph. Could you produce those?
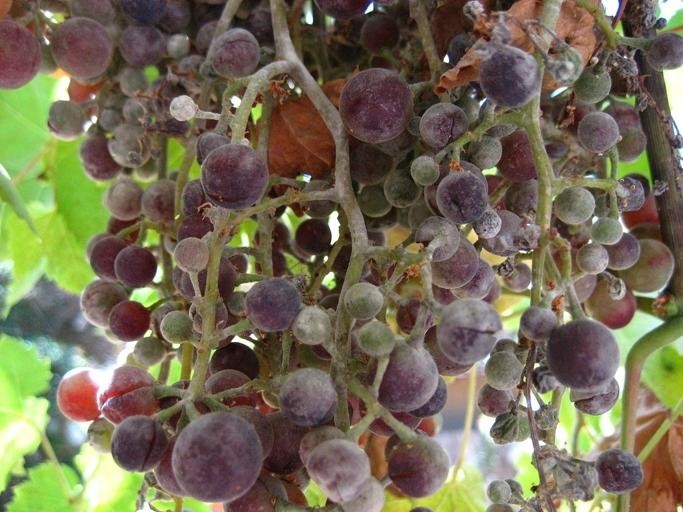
[0,0,683,512]
